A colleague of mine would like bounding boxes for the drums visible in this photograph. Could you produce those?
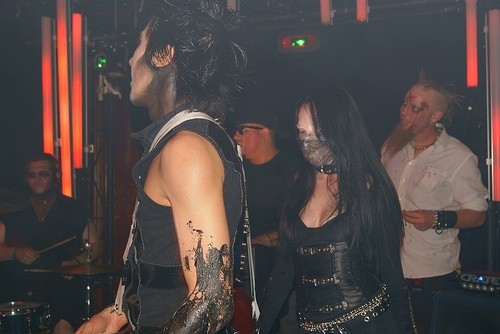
[0,301,52,334]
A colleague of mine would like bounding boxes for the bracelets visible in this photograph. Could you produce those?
[73,257,81,264]
[12,250,17,260]
[432,210,457,230]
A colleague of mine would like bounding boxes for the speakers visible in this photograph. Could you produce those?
[430,290,500,334]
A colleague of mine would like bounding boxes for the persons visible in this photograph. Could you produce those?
[74,0,245,334]
[0,153,103,334]
[381,80,488,334]
[257,86,414,334]
[234,102,294,334]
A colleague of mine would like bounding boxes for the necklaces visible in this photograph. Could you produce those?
[315,165,337,174]
[410,138,437,150]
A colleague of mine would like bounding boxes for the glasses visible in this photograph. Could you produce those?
[237,124,263,134]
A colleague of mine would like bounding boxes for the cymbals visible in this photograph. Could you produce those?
[0,188,31,214]
[55,263,118,275]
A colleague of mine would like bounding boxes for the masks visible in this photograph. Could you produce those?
[298,133,335,166]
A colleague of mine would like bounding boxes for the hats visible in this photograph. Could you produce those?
[227,85,283,131]
